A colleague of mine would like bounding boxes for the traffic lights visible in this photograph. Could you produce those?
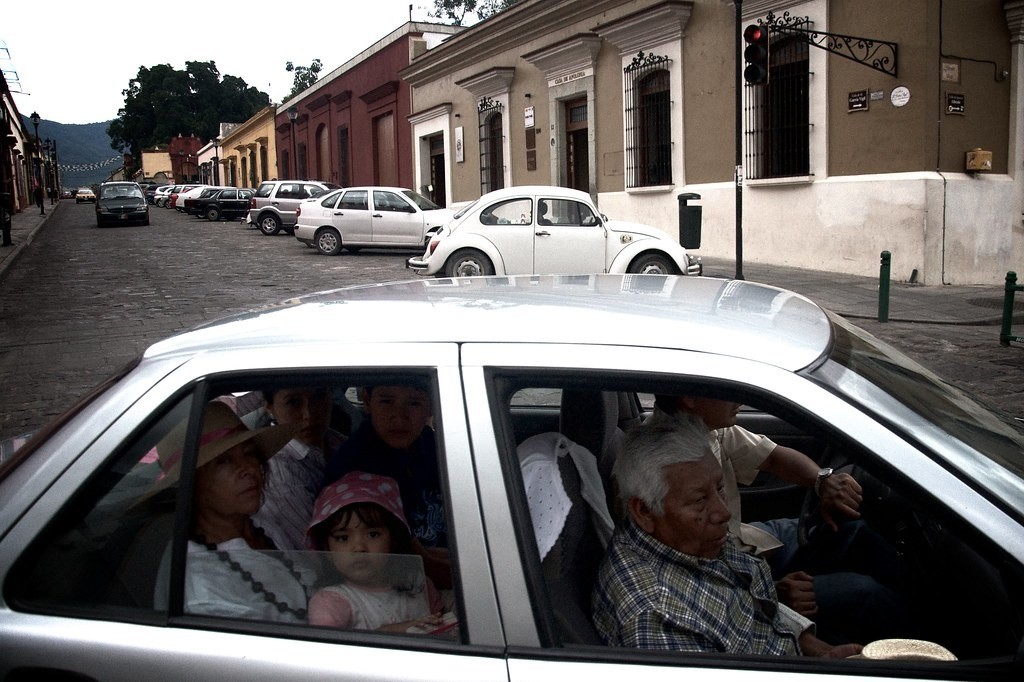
[744,23,768,86]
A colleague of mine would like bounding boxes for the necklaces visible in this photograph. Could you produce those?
[197,517,310,617]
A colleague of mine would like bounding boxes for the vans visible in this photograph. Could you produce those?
[63,189,72,199]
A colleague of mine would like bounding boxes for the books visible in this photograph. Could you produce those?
[405,611,458,636]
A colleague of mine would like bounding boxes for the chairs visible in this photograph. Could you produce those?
[538,200,554,226]
[105,506,177,615]
[514,384,635,651]
[378,199,386,208]
[106,185,135,195]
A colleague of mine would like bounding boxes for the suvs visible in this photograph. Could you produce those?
[249,178,345,236]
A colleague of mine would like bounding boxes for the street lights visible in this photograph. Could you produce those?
[286,104,299,180]
[29,111,47,217]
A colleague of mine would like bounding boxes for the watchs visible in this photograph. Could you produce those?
[815,468,834,498]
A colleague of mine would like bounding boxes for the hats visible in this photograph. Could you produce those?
[302,471,411,553]
[121,401,297,518]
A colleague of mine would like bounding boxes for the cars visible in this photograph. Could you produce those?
[76,188,97,204]
[294,186,455,256]
[140,182,258,222]
[0,272,1024,682]
[407,185,707,277]
[95,179,150,227]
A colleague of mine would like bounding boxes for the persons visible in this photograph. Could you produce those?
[34,187,42,207]
[640,389,897,633]
[589,416,864,662]
[135,375,461,641]
